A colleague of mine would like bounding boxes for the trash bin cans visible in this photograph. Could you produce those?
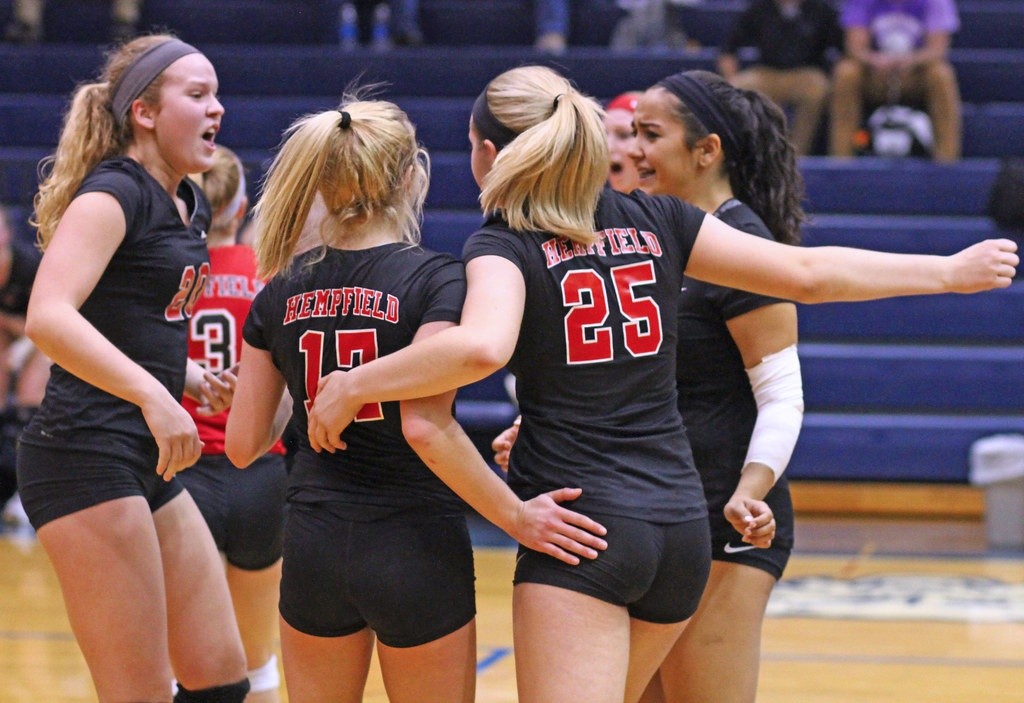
[973,438,1024,545]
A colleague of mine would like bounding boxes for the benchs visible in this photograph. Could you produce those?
[0,0,1024,514]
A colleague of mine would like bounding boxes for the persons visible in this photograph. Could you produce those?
[0,0,1021,703]
[827,0,963,163]
